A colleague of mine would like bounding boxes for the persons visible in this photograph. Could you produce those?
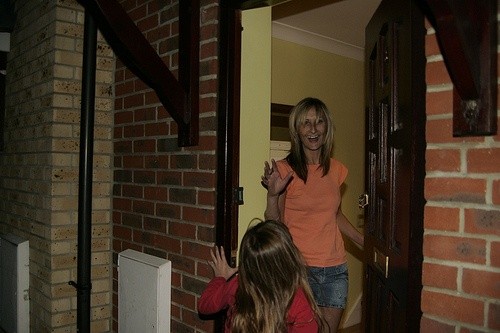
[260,96,365,333]
[197,218,325,333]
